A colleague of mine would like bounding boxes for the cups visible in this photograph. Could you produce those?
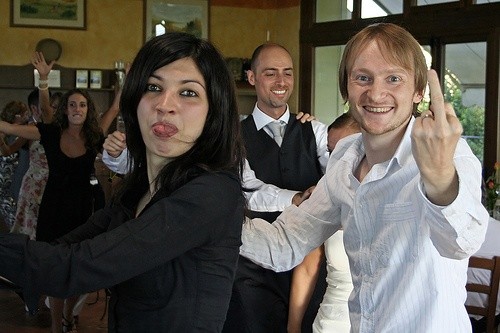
[114,62,126,88]
[116,116,125,135]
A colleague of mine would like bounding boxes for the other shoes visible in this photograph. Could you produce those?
[72,315,79,333]
[62,316,74,333]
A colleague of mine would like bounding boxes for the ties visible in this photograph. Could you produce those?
[266,120,285,148]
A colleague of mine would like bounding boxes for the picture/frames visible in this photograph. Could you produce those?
[10,0,87,30]
[145,2,211,44]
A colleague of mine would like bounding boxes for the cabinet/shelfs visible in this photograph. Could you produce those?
[0,65,119,134]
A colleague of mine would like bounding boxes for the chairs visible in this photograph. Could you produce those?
[465,256,500,333]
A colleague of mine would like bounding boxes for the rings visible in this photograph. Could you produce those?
[422,114,434,120]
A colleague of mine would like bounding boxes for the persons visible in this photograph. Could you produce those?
[287,112,369,332]
[0,31,260,333]
[237,21,490,333]
[463,214,499,333]
[102,130,316,212]
[231,42,331,332]
[0,50,131,333]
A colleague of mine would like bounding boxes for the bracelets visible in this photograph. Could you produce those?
[38,78,49,90]
